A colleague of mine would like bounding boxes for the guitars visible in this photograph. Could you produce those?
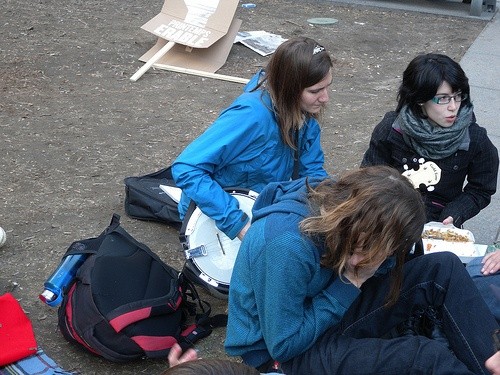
[179,188,258,293]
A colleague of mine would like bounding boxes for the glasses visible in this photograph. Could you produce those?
[432,90,468,104]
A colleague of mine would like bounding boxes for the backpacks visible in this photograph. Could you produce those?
[39,213,228,363]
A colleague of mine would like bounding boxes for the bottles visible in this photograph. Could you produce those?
[39,242,92,307]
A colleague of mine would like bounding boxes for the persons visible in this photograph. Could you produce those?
[161,343,260,375]
[360,53,500,229]
[170,34,333,301]
[224,164,500,375]
[465,231,500,318]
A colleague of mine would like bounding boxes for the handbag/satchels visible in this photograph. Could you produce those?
[123,164,180,221]
